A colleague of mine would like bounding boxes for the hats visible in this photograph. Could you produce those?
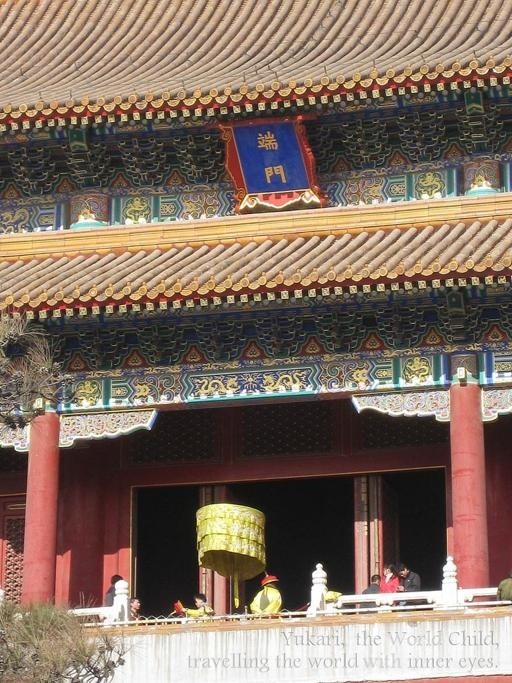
[173,599,185,616]
[260,575,279,587]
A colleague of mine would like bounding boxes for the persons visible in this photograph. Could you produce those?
[249,574,285,614]
[380,562,400,611]
[130,596,143,625]
[358,573,381,613]
[395,563,421,611]
[105,574,124,606]
[174,593,217,620]
